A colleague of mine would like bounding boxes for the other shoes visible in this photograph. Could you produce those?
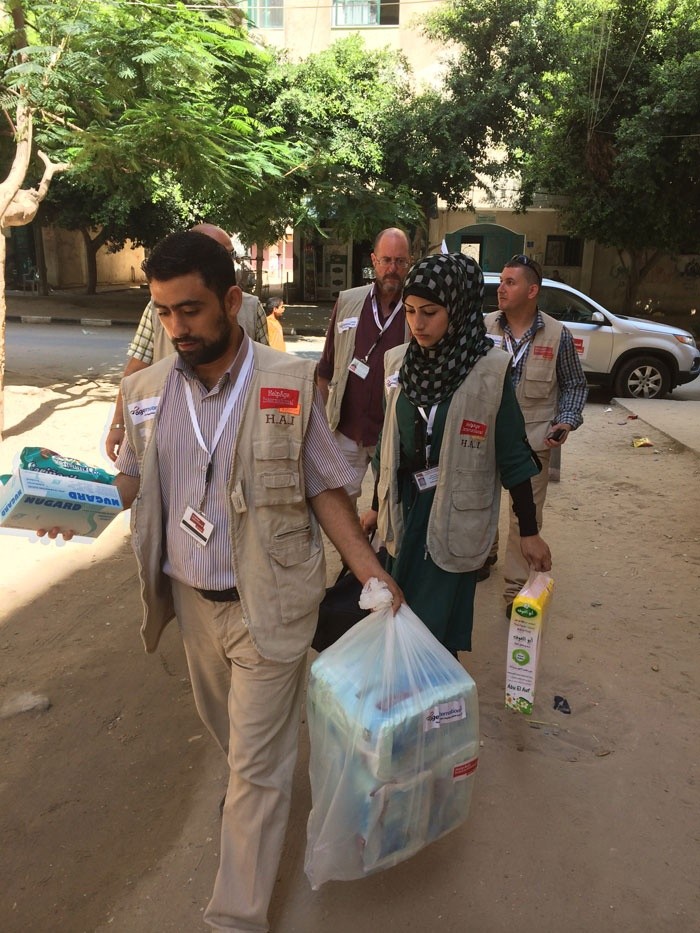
[475,553,498,583]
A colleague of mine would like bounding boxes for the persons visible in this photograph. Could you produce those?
[315,225,419,507]
[265,295,288,353]
[104,222,269,465]
[351,250,554,653]
[29,230,408,933]
[483,254,590,617]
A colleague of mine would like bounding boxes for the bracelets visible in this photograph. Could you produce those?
[110,422,126,432]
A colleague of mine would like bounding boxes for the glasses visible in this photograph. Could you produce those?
[375,255,411,269]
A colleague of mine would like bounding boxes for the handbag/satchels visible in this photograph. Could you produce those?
[311,529,393,651]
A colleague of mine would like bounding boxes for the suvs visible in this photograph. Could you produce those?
[481,270,699,399]
